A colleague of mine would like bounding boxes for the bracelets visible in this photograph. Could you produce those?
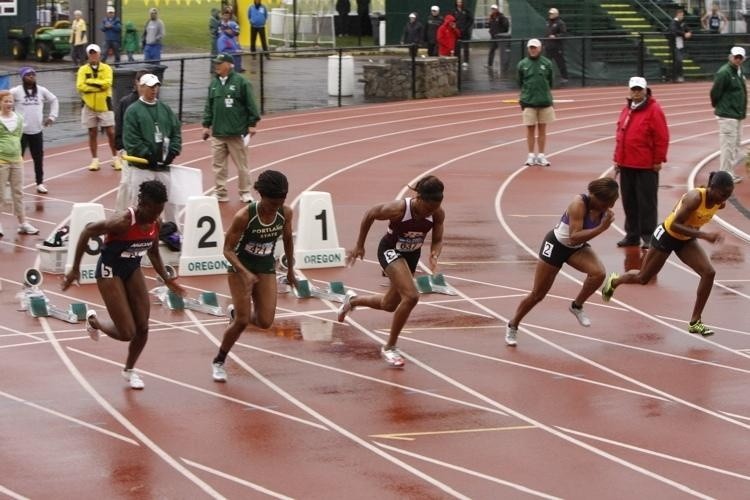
[433,255,438,259]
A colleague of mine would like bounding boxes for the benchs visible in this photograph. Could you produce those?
[508,0,711,82]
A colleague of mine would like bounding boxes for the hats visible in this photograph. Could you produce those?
[212,52,235,64]
[491,4,499,10]
[226,22,241,34]
[628,76,647,88]
[106,6,115,12]
[431,6,440,11]
[18,66,37,79]
[548,8,558,15]
[86,44,100,55]
[527,38,543,49]
[731,47,746,58]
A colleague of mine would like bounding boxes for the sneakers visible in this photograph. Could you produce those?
[524,156,536,166]
[211,193,230,203]
[642,242,650,249]
[505,319,518,346]
[568,301,591,328]
[139,73,161,87]
[121,366,145,389]
[85,309,101,342]
[36,184,48,194]
[17,223,40,235]
[380,346,405,368]
[688,318,714,336]
[535,155,551,166]
[226,304,236,326]
[89,160,101,171]
[618,235,640,246]
[240,193,253,203]
[338,289,358,322]
[111,159,120,169]
[210,358,227,383]
[601,272,620,303]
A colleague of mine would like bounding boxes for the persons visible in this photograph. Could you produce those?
[0,90,39,237]
[514,38,556,168]
[336,176,444,367]
[699,0,728,34]
[666,9,693,84]
[69,10,88,67]
[207,0,246,74]
[122,22,140,62]
[483,4,510,70]
[201,53,261,202]
[143,8,165,65]
[62,180,186,390]
[210,169,300,382]
[114,69,182,221]
[248,0,271,60]
[399,0,474,67]
[709,46,750,184]
[99,6,122,63]
[543,7,569,84]
[358,1,372,38]
[8,66,58,193]
[601,171,735,337]
[613,76,670,249]
[504,177,619,347]
[336,0,353,37]
[77,44,122,170]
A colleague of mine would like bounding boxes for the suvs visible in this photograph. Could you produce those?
[7,19,72,63]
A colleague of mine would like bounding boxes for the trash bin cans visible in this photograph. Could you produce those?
[495,33,511,78]
[112,63,168,150]
[368,10,386,45]
